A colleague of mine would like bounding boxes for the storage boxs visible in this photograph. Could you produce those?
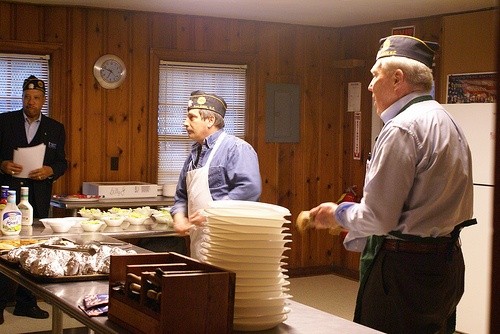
[82,180,158,198]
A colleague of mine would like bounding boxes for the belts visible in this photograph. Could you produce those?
[381,240,460,254]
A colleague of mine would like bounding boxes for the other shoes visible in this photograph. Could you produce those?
[0,308,4,324]
[13,304,50,319]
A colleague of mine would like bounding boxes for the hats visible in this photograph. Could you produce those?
[22,75,45,94]
[376,35,440,69]
[187,90,228,118]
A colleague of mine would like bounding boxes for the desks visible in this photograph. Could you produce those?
[0,216,388,334]
[48,196,176,217]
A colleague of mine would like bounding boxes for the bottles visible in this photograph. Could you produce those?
[17,187,33,226]
[0,190,22,235]
[0,185,12,231]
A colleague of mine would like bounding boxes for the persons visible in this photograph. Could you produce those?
[0,75,68,325]
[309,35,477,334]
[169,91,262,262]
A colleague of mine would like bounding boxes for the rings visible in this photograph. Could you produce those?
[12,171,14,174]
[38,176,41,180]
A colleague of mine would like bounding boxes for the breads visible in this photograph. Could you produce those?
[7,239,137,278]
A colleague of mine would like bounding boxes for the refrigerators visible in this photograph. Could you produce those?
[433,101,500,334]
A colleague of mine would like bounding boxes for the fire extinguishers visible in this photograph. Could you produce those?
[339,185,359,237]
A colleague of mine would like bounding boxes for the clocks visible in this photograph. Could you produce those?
[93,54,127,90]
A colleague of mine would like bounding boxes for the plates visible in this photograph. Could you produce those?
[197,199,293,331]
[38,212,175,233]
